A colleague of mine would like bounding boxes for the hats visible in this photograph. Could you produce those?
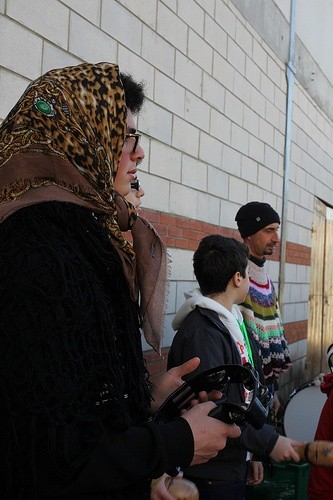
[235,201,280,238]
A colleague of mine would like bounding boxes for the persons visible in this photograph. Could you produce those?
[166,235,307,500]
[236,201,292,381]
[0,61,240,500]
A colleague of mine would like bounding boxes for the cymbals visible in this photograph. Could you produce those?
[151,363,274,432]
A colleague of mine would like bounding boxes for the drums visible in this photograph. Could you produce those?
[281,376,328,440]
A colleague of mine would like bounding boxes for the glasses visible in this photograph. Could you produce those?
[130,179,141,191]
[126,134,141,153]
